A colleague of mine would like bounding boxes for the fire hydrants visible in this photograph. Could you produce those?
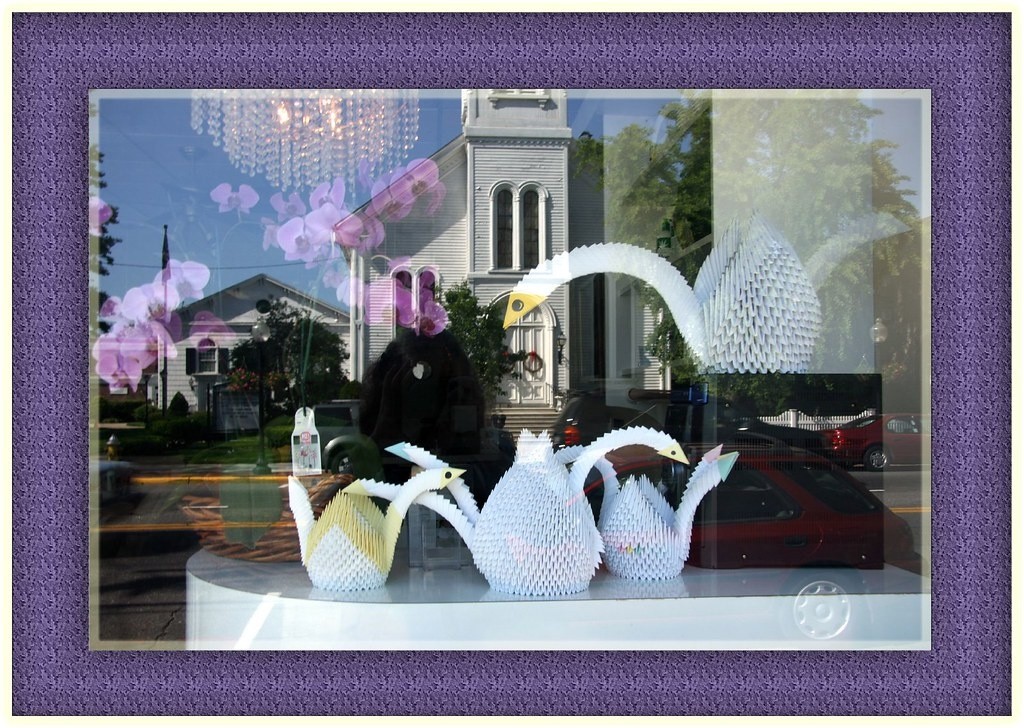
[107,434,122,461]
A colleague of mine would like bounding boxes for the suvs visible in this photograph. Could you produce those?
[548,386,840,469]
[300,399,516,481]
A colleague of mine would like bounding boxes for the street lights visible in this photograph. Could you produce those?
[252,315,272,476]
[870,318,888,414]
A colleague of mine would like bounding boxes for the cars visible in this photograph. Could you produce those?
[99,461,147,523]
[508,444,929,641]
[815,412,922,472]
